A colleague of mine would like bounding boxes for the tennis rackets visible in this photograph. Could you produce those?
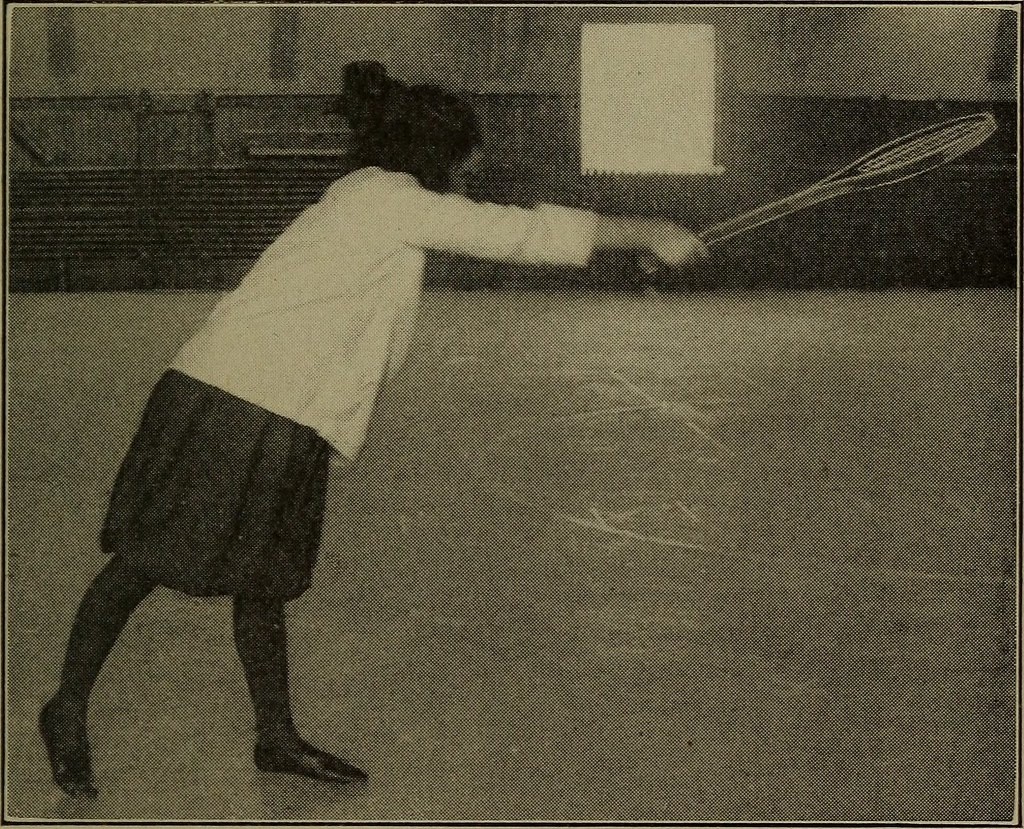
[636,110,999,276]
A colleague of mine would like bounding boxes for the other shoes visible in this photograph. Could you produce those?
[37,700,99,800]
[254,739,366,785]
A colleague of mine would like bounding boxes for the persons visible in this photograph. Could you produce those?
[37,60,712,798]
